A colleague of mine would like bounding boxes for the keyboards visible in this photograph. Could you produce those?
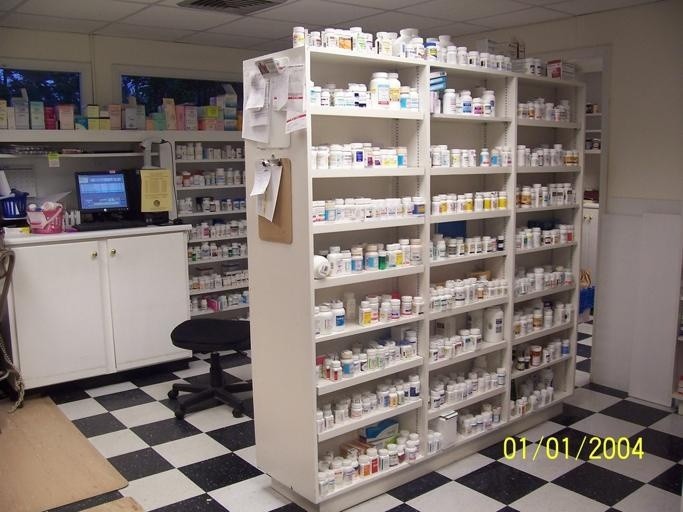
[74,220,147,231]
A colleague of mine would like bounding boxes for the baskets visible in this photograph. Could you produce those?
[0,189,29,218]
[26,208,63,233]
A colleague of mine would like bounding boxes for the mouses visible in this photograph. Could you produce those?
[172,218,182,225]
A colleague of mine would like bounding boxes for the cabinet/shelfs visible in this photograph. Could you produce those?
[239,48,602,512]
[1,126,246,393]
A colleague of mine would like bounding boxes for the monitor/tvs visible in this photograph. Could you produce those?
[75,170,131,222]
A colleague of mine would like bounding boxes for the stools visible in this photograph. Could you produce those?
[165,317,251,424]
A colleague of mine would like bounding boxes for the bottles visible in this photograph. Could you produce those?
[313,237,422,281]
[175,143,249,312]
[309,70,421,113]
[319,428,420,497]
[518,143,578,168]
[428,350,507,413]
[514,299,576,336]
[426,428,443,453]
[516,182,578,209]
[442,86,499,116]
[313,194,427,227]
[322,323,418,383]
[429,274,509,313]
[314,290,426,337]
[292,26,548,77]
[458,399,504,439]
[317,371,420,435]
[515,334,573,371]
[510,366,560,420]
[515,262,574,296]
[430,188,508,215]
[429,143,511,169]
[515,223,577,252]
[429,233,507,265]
[518,98,572,123]
[314,139,410,169]
[430,304,509,364]
